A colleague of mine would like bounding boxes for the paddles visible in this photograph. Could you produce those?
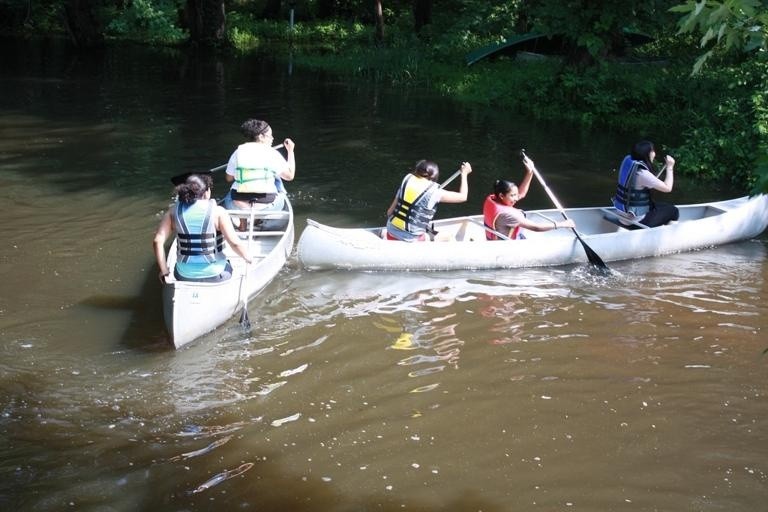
[170,140,289,187]
[521,150,608,270]
[239,199,257,334]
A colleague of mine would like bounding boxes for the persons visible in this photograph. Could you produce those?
[612,142,680,231]
[385,158,473,246]
[152,172,255,287]
[224,119,297,219]
[482,156,576,242]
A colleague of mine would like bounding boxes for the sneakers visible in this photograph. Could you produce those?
[235,227,247,240]
